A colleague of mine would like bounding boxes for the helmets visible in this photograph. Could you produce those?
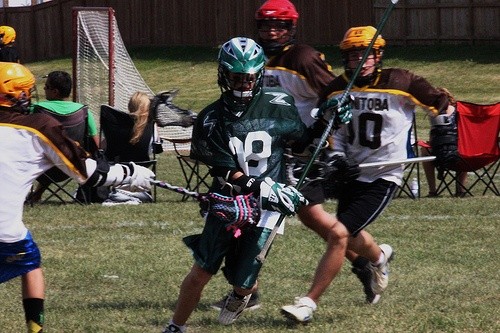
[0,25,16,48]
[255,0,300,56]
[218,37,266,107]
[0,61,37,107]
[339,26,387,83]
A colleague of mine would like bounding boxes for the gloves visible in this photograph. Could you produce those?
[429,126,459,163]
[260,176,310,217]
[128,162,156,191]
[328,154,361,182]
[318,94,355,131]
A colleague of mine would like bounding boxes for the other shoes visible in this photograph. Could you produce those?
[455,192,466,196]
[425,192,438,197]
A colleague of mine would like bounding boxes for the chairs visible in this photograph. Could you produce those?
[392,100,500,200]
[162,136,211,202]
[30,105,158,208]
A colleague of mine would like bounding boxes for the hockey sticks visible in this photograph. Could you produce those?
[218,0,400,326]
[284,155,438,186]
[154,102,305,164]
[150,179,262,230]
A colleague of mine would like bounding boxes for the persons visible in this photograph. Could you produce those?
[255,0,380,305]
[0,62,156,333]
[30,71,99,201]
[420,87,466,198]
[164,36,355,332]
[99,92,159,144]
[0,26,20,62]
[280,26,452,324]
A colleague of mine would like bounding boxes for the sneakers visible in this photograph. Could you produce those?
[219,288,252,325]
[369,244,395,294]
[211,289,260,310]
[164,324,184,333]
[351,256,381,305]
[279,304,313,323]
